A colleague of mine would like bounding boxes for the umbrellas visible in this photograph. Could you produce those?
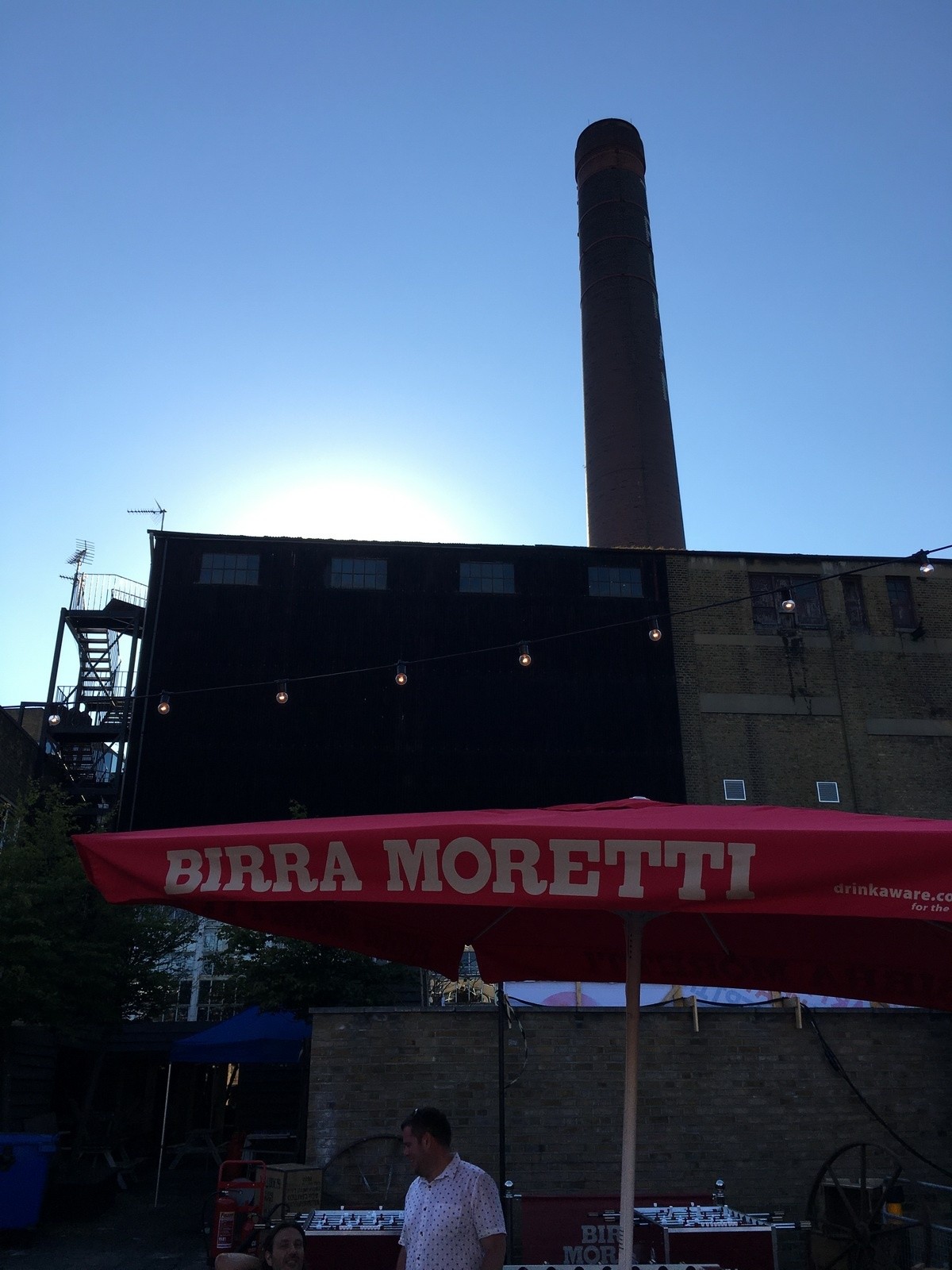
[73,793,952,1270]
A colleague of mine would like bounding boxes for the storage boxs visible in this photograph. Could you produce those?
[254,1163,324,1219]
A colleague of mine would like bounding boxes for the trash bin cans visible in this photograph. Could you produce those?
[0,1133,60,1248]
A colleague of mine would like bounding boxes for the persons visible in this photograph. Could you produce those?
[263,1222,306,1270]
[395,1107,506,1270]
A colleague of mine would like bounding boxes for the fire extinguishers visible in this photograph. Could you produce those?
[211,1189,243,1259]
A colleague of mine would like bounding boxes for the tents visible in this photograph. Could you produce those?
[154,1005,312,1208]
